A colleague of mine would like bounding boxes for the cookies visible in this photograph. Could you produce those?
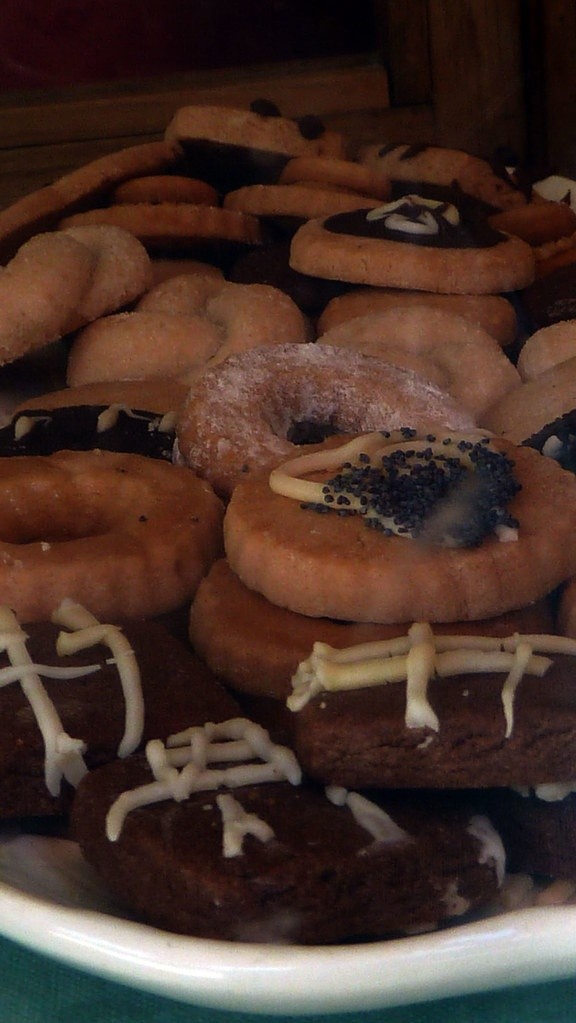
[0,96,576,948]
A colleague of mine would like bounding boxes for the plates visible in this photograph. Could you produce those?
[1,1,575,1015]
